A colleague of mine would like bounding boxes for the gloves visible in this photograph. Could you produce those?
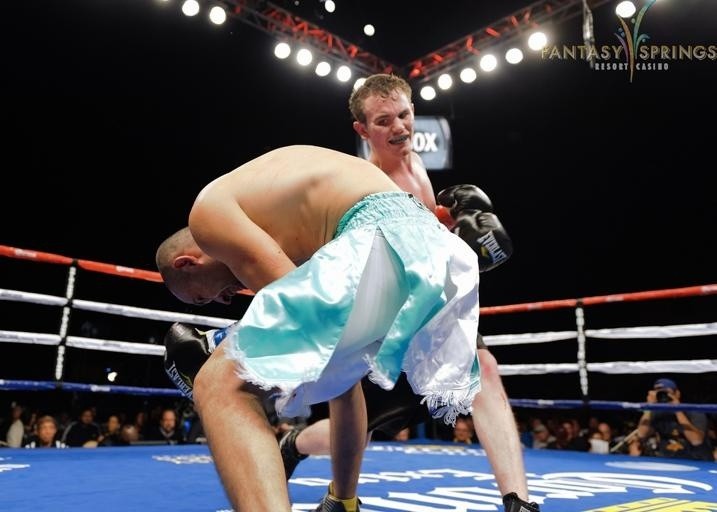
[436,184,513,273]
[163,322,238,407]
[276,427,310,481]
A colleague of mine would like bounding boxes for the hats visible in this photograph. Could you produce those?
[654,379,677,391]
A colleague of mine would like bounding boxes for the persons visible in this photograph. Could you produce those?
[156,144,479,512]
[373,378,717,460]
[1,395,207,449]
[276,74,540,512]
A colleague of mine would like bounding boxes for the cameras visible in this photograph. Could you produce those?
[655,391,670,403]
